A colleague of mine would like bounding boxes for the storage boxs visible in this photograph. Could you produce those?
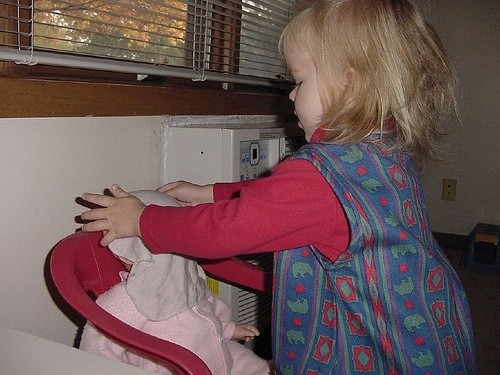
[473,223,500,273]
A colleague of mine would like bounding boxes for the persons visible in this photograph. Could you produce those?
[82,1,477,375]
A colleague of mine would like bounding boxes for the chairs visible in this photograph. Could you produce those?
[50,229,274,375]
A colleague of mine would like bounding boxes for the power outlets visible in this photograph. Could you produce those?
[441,177,457,201]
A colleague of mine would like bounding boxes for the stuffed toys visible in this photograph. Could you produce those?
[80,190,271,375]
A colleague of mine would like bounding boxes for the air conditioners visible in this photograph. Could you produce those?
[165,121,311,362]
